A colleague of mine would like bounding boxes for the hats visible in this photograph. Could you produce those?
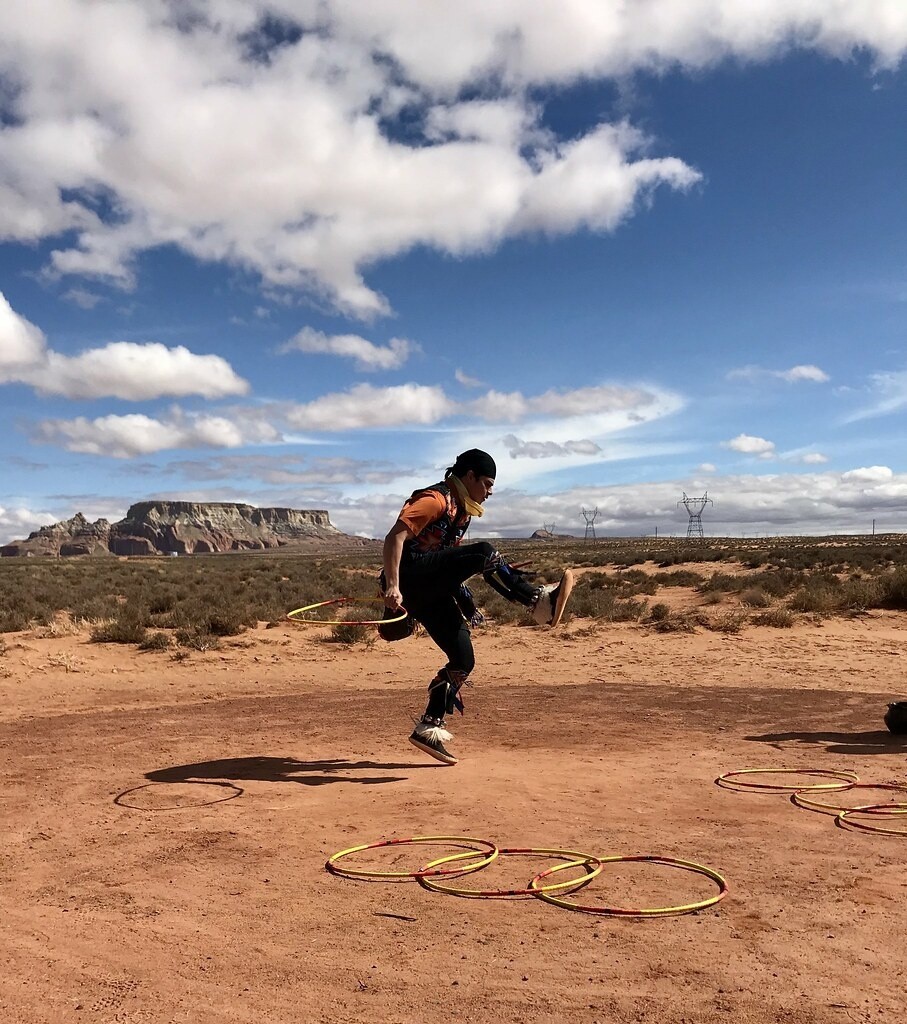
[456,449,496,479]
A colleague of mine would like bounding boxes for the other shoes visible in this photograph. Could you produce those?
[408,721,458,764]
[533,569,573,628]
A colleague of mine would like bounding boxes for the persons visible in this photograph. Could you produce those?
[383,449,573,764]
